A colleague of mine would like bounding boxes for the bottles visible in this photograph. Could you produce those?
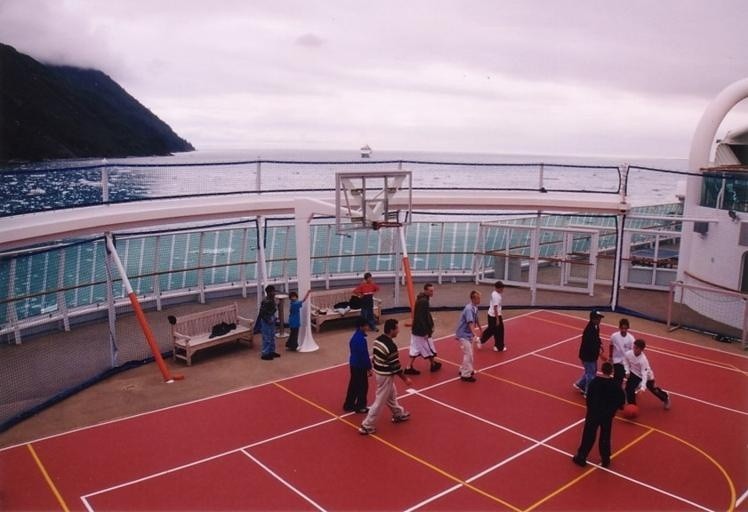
[590,309,604,318]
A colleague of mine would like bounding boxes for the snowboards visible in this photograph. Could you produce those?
[623,404,639,418]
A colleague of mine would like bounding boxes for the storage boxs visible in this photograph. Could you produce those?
[310,287,382,333]
[171,301,254,366]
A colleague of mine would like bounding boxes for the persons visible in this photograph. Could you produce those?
[623,339,671,409]
[359,319,413,434]
[343,319,374,414]
[573,308,608,399]
[404,283,442,374]
[572,362,625,468]
[608,317,636,386]
[475,280,507,352]
[353,272,379,332]
[258,285,280,360]
[455,288,482,382]
[285,289,312,350]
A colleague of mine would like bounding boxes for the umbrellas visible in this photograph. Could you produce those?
[270,352,280,357]
[261,355,272,360]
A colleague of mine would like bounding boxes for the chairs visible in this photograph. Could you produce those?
[392,412,409,421]
[475,336,482,350]
[404,368,420,375]
[358,425,376,433]
[355,407,369,412]
[583,391,588,398]
[572,455,587,466]
[430,361,441,371]
[461,376,476,381]
[663,391,671,409]
[493,346,507,352]
[572,381,585,393]
